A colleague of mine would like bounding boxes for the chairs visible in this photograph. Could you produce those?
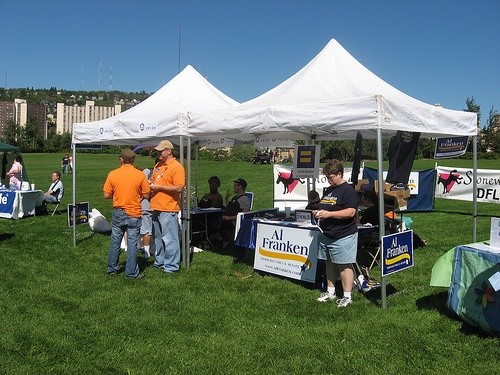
[368,217,395,271]
[41,189,65,215]
[212,191,255,247]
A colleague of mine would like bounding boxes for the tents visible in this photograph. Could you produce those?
[72,38,479,308]
[0,142,31,189]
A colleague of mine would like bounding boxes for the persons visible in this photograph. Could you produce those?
[61,153,73,175]
[148,140,185,273]
[312,159,358,307]
[121,169,153,258]
[354,190,379,245]
[6,156,23,187]
[306,189,318,212]
[103,149,151,279]
[210,178,251,243]
[189,176,223,237]
[41,170,64,203]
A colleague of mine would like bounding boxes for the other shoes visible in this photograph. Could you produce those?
[337,296,352,307]
[127,273,145,280]
[108,272,116,277]
[316,292,336,303]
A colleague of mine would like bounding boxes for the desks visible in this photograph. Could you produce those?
[0,189,44,219]
[430,240,500,336]
[188,209,221,251]
[234,216,378,291]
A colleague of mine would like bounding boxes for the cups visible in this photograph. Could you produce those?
[285,206,291,218]
[30,183,35,191]
[224,190,233,201]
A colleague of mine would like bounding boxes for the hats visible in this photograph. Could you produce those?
[233,178,248,187]
[153,140,173,151]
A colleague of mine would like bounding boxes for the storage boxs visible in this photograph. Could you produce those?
[356,179,411,213]
